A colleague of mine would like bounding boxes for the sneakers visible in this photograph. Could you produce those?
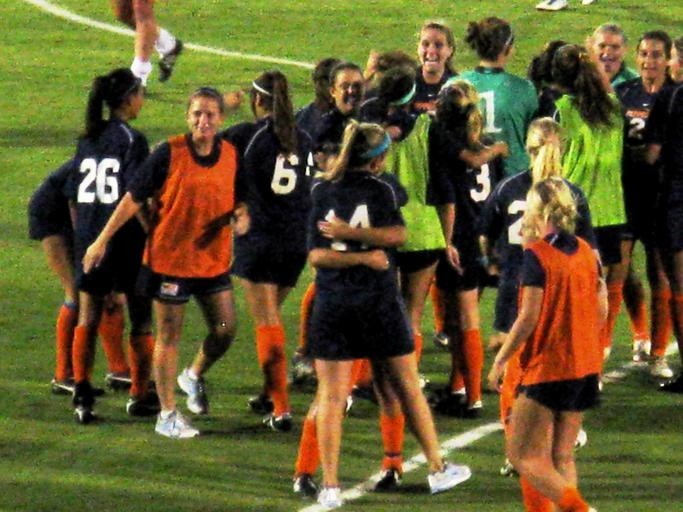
[286,474,342,508]
[175,369,211,415]
[291,350,317,393]
[370,462,404,491]
[152,408,198,440]
[246,392,293,431]
[158,36,181,82]
[432,328,452,355]
[49,376,104,420]
[104,370,161,416]
[437,384,484,418]
[633,340,683,393]
[426,462,474,496]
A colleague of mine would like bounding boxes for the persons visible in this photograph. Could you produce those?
[535,0,594,11]
[110,1,182,94]
[68,65,157,420]
[215,68,309,436]
[81,85,246,439]
[27,154,154,395]
[293,16,682,511]
